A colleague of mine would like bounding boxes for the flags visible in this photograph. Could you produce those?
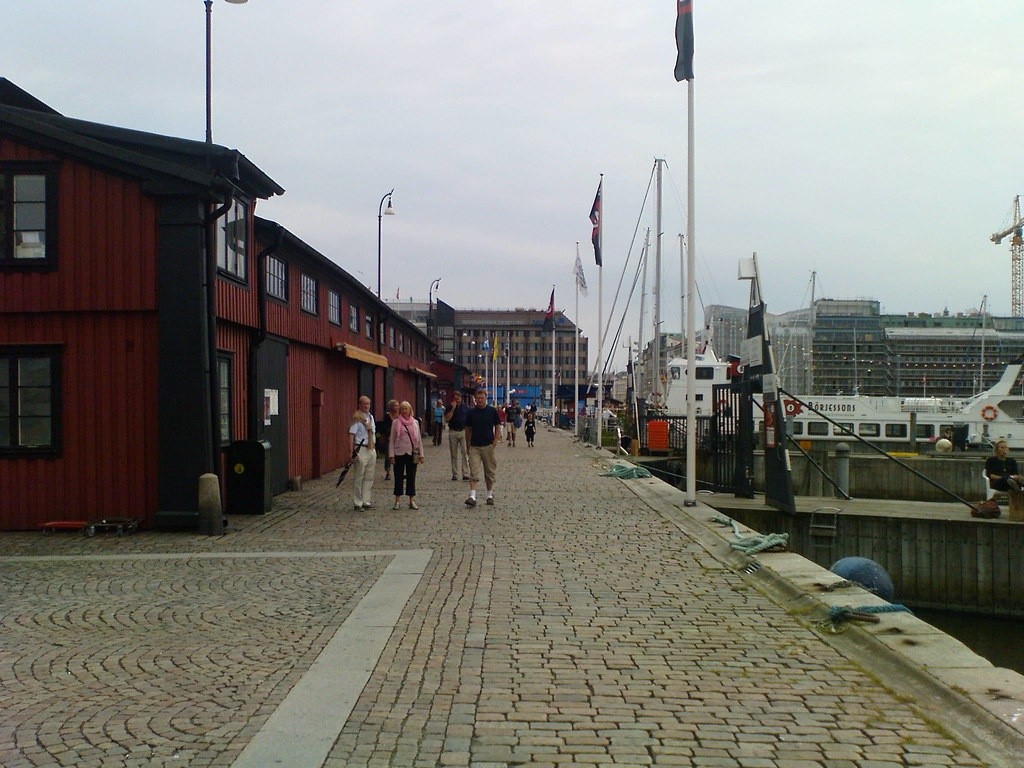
[542,289,554,333]
[481,333,490,352]
[572,246,589,297]
[503,337,509,358]
[675,0,696,82]
[493,336,498,362]
[589,180,602,267]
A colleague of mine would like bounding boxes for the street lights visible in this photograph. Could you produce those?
[430,278,443,340]
[377,188,396,296]
[202,0,249,514]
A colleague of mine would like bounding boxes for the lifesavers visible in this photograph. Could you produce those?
[981,405,999,421]
[648,392,664,408]
[717,400,729,413]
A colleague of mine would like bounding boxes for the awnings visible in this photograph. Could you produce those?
[408,364,437,378]
[337,343,389,368]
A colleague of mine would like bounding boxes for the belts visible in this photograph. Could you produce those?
[354,443,368,447]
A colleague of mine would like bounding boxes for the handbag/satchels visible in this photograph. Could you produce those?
[412,449,420,464]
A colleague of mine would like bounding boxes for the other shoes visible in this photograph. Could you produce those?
[531,443,534,447]
[487,498,494,505]
[410,501,419,510]
[528,443,531,447]
[463,476,479,481]
[362,502,377,509]
[508,442,511,447]
[353,506,365,512]
[452,475,458,480]
[466,497,477,506]
[394,501,399,510]
[513,442,516,447]
[386,473,392,480]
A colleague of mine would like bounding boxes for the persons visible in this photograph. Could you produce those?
[465,389,501,507]
[579,407,588,416]
[525,412,536,448]
[524,401,537,412]
[376,399,400,481]
[444,391,470,482]
[432,397,445,446]
[983,440,1024,508]
[490,399,523,448]
[347,396,377,514]
[389,401,425,511]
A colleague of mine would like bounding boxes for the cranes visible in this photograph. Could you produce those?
[990,195,1024,319]
